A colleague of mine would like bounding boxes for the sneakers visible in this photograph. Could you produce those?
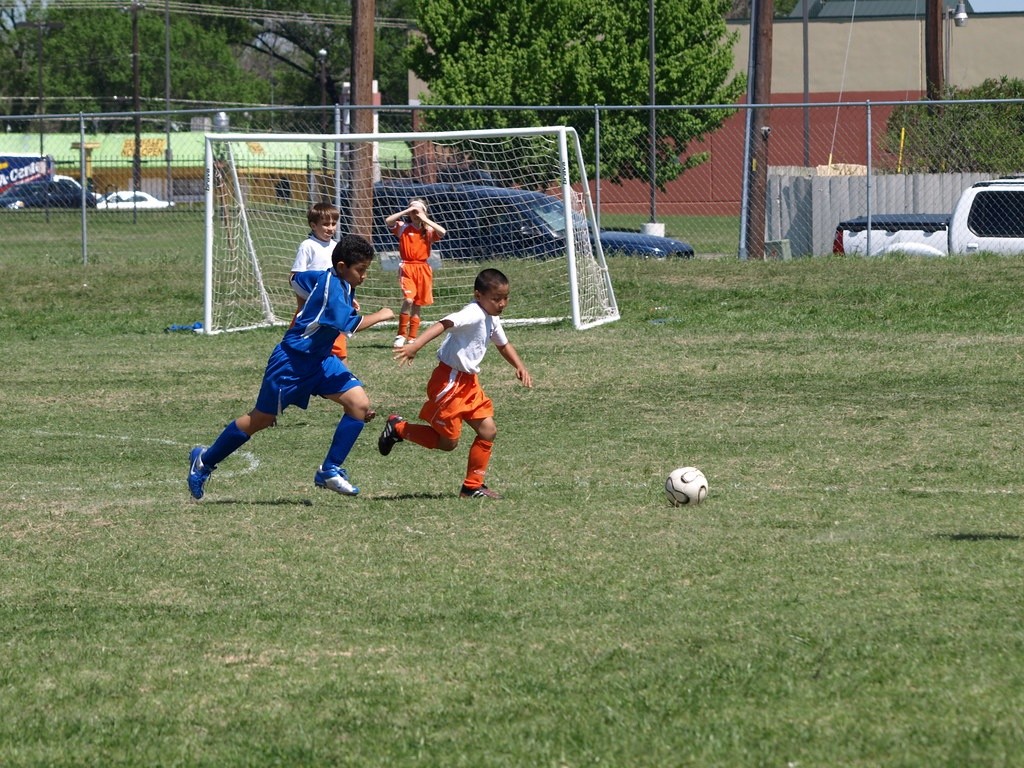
[187,445,217,499]
[407,338,415,343]
[393,334,406,348]
[378,414,404,456]
[314,464,359,496]
[459,484,504,501]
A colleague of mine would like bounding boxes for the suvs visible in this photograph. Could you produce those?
[335,176,697,265]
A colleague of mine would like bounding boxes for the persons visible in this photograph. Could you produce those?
[378,268,532,500]
[187,235,395,500]
[290,203,376,423]
[385,198,446,347]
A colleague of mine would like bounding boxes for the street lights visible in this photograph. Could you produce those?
[318,47,330,204]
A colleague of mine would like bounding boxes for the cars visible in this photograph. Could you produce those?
[96,190,175,211]
[0,179,98,210]
[53,174,103,207]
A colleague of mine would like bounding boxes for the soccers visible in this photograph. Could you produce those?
[666,466,708,507]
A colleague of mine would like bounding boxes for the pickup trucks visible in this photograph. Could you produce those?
[830,175,1024,259]
